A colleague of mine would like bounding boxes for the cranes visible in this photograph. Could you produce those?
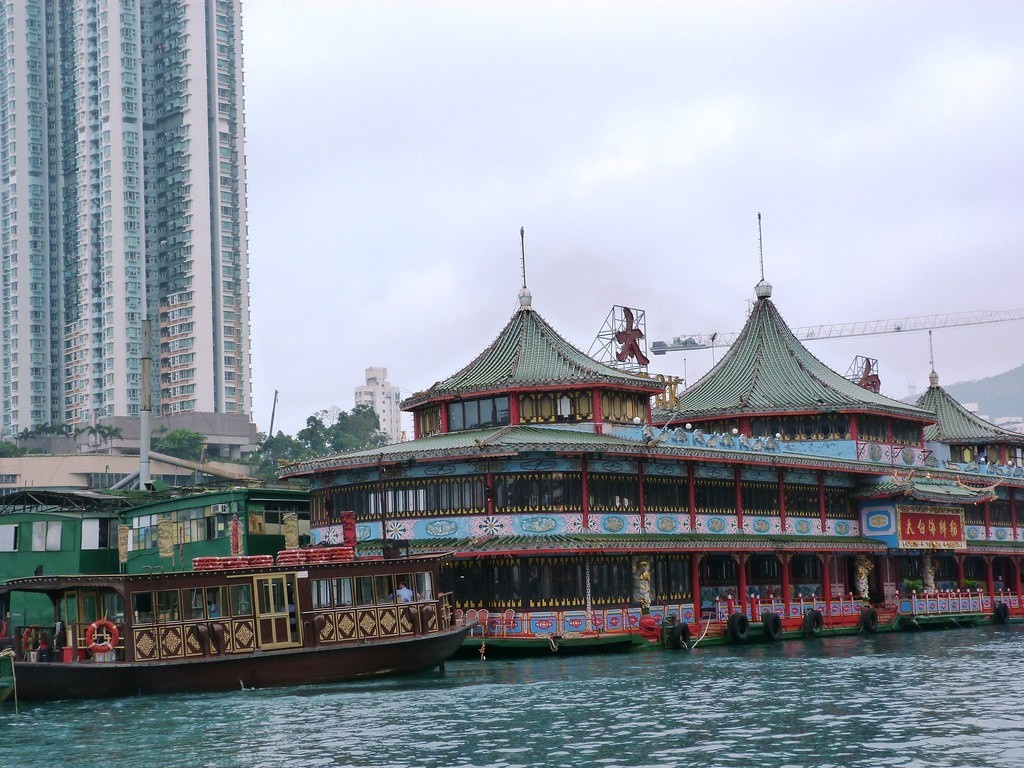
[648,305,1024,356]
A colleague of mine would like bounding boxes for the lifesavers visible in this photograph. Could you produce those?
[865,609,878,632]
[763,613,783,641]
[997,603,1010,625]
[808,610,824,633]
[277,546,355,563]
[729,613,751,641]
[85,619,119,652]
[22,624,46,653]
[671,623,691,649]
[192,555,276,569]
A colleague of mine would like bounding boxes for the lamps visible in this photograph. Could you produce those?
[732,428,738,434]
[633,417,641,424]
[981,457,985,463]
[685,423,692,430]
[775,433,781,439]
[1007,461,1013,466]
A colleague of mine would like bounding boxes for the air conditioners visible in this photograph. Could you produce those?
[211,504,230,514]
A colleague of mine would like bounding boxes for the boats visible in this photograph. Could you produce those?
[0,545,478,708]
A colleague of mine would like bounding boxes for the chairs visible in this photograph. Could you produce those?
[494,608,515,638]
[454,609,488,637]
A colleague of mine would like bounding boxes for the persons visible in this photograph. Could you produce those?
[388,581,421,602]
